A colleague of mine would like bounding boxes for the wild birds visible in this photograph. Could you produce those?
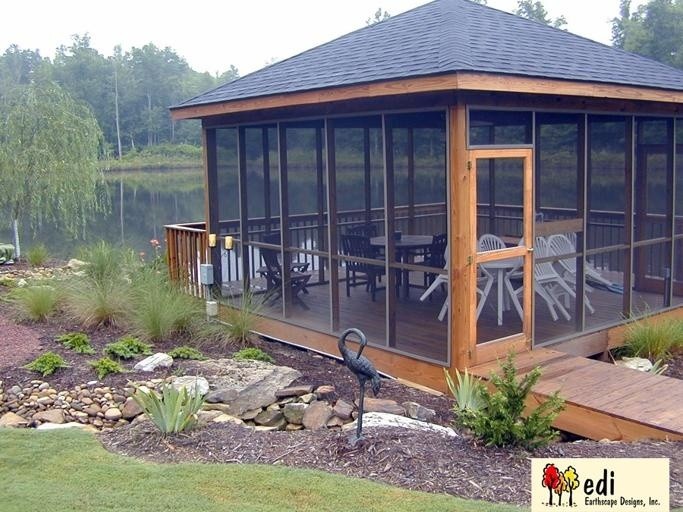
[338,327,381,439]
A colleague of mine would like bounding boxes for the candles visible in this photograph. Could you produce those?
[208,233,216,246]
[224,235,232,248]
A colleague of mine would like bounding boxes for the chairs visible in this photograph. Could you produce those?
[263,233,310,294]
[408,233,447,300]
[339,234,399,302]
[346,224,381,287]
[257,240,312,311]
[417,230,611,324]
